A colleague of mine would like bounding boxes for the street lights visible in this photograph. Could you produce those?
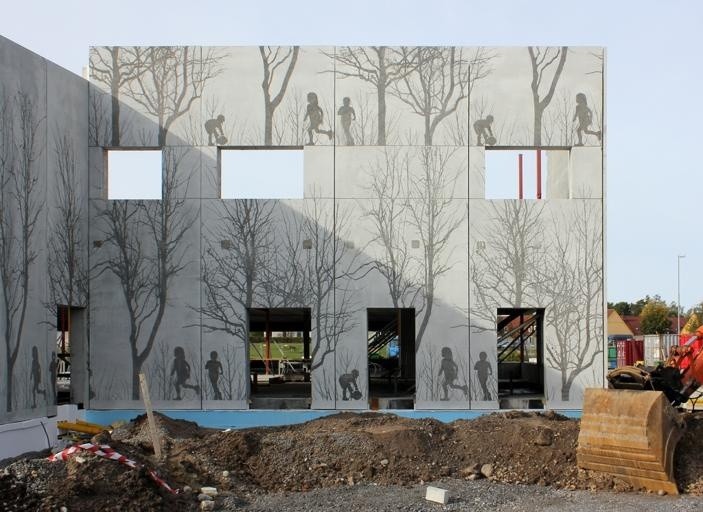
[677,254,686,346]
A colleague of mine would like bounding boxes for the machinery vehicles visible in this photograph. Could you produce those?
[576,325,703,495]
